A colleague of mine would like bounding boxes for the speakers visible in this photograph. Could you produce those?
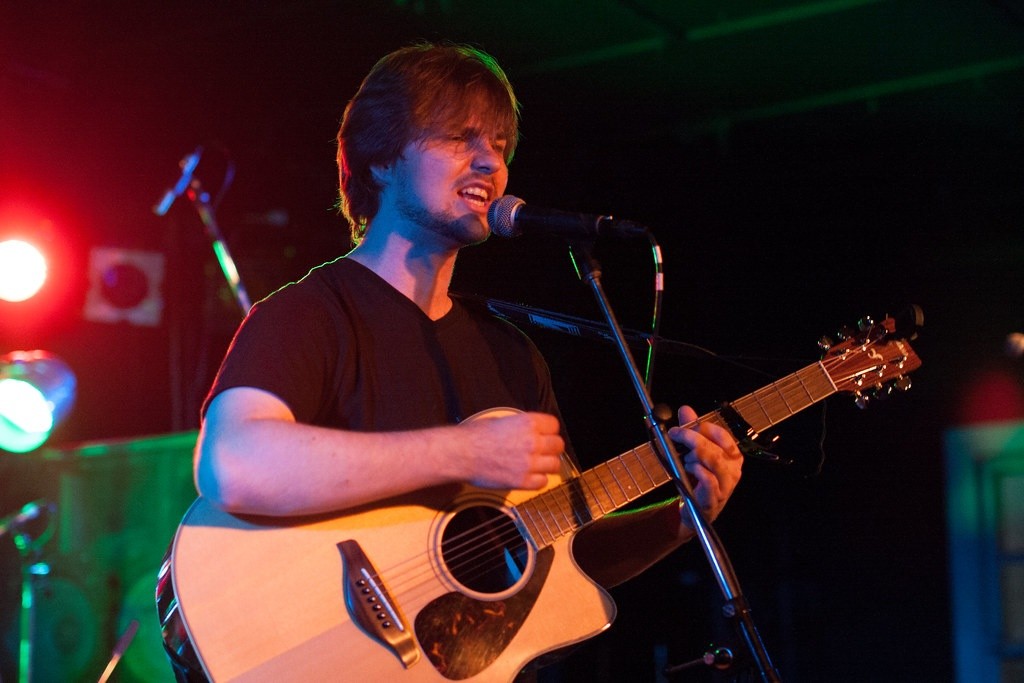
[0,430,199,683]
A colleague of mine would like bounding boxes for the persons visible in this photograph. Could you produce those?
[192,43,743,683]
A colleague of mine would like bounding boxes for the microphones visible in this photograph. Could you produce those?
[0,498,51,536]
[1004,333,1024,358]
[488,194,647,239]
[152,146,204,217]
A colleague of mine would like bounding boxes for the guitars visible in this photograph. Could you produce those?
[154,300,925,683]
